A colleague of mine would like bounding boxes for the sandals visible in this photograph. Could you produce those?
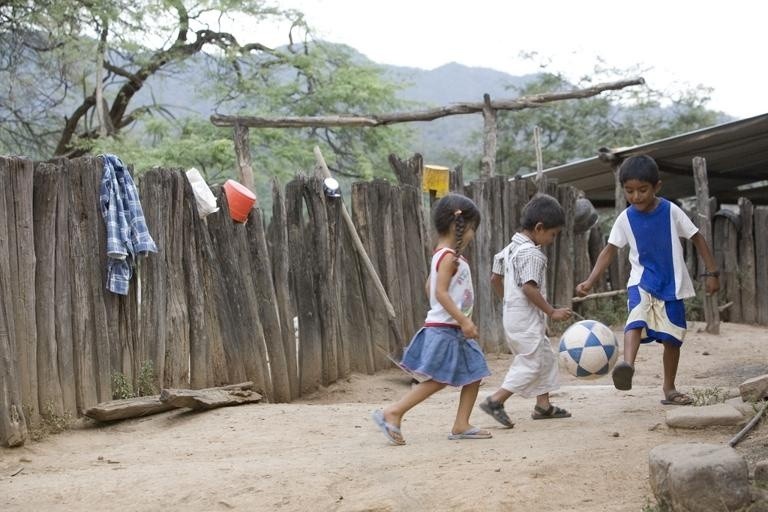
[530,399,575,420]
[481,396,515,428]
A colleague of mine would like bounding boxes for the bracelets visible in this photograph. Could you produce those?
[705,270,720,277]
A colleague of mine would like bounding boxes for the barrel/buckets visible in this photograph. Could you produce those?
[222,179,257,224]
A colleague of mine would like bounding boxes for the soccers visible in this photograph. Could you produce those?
[559,318,618,382]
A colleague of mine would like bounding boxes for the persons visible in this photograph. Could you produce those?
[373,192,493,445]
[479,194,573,427]
[572,152,720,406]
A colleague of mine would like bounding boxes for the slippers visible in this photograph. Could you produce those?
[372,407,407,448]
[660,391,694,405]
[447,424,493,440]
[611,358,635,391]
